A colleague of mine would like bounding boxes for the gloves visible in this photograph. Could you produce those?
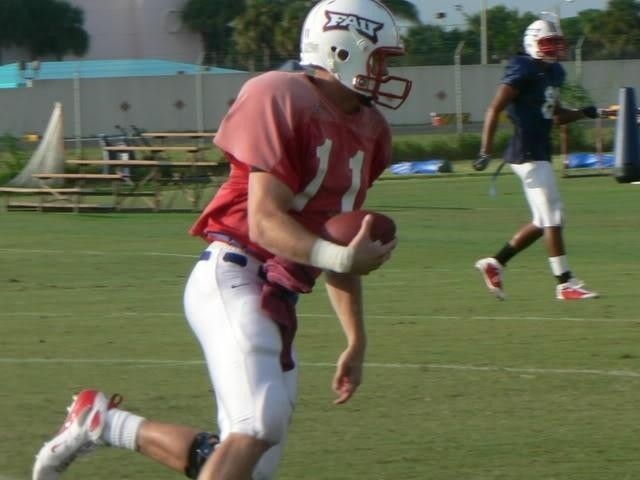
[583,105,602,119]
[473,155,489,172]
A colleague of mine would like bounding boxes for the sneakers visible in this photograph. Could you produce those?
[555,278,598,300]
[30,388,124,479]
[474,257,507,302]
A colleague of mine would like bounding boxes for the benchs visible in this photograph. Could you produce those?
[0,132,231,213]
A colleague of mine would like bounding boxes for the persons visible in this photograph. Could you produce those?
[470,18,597,299]
[29,0,411,479]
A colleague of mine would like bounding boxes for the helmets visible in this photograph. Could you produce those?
[300,0,406,96]
[523,20,565,63]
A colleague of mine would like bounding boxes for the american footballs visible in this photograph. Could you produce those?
[323,210,396,245]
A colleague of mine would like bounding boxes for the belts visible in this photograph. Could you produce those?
[199,250,267,282]
